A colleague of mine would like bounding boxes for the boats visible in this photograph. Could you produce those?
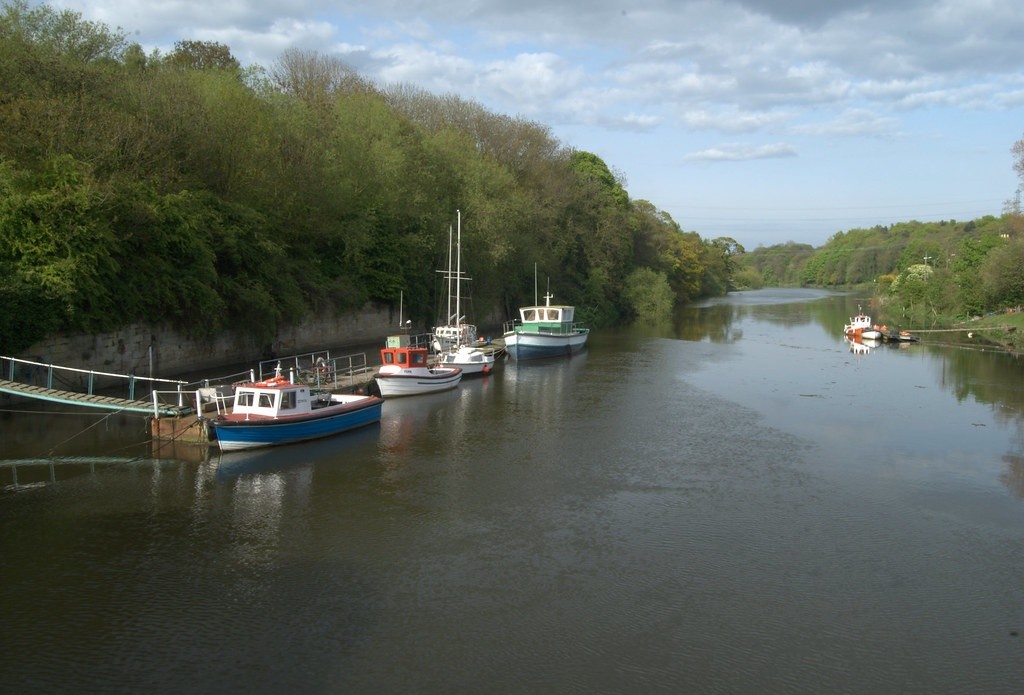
[208,374,386,452]
[502,262,590,360]
[373,345,463,398]
[844,304,882,340]
[844,335,882,354]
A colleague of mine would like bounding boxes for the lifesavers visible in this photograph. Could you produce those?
[254,373,291,387]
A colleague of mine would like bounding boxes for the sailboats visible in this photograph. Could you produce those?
[426,222,489,354]
[431,210,496,374]
